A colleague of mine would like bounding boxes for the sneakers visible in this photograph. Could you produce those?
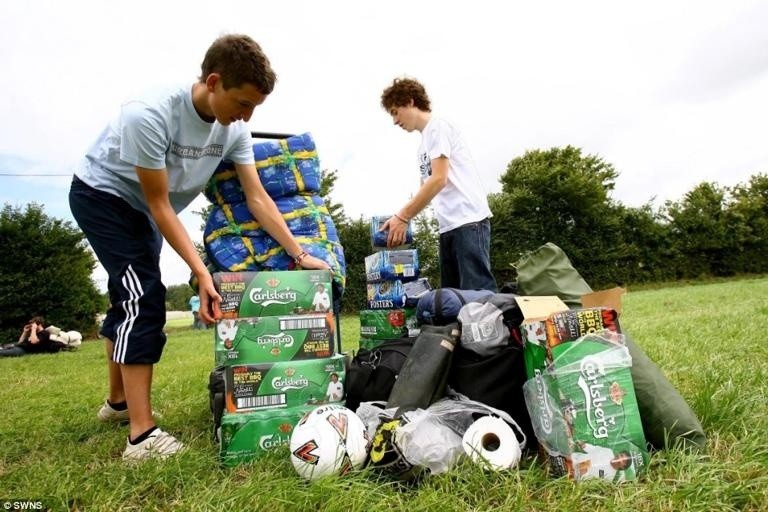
[97,399,164,424]
[122,431,186,463]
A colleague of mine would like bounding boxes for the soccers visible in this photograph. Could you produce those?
[289,405,370,483]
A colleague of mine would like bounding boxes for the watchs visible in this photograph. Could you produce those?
[295,251,308,265]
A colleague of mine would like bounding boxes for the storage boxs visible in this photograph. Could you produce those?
[512,285,651,485]
[207,267,420,467]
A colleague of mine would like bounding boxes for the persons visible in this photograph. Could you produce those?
[0,316,49,357]
[571,440,632,484]
[188,293,207,331]
[216,318,241,350]
[312,283,331,311]
[378,77,502,296]
[394,214,409,225]
[68,34,338,462]
[322,372,344,403]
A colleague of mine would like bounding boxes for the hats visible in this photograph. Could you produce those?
[346,337,540,460]
